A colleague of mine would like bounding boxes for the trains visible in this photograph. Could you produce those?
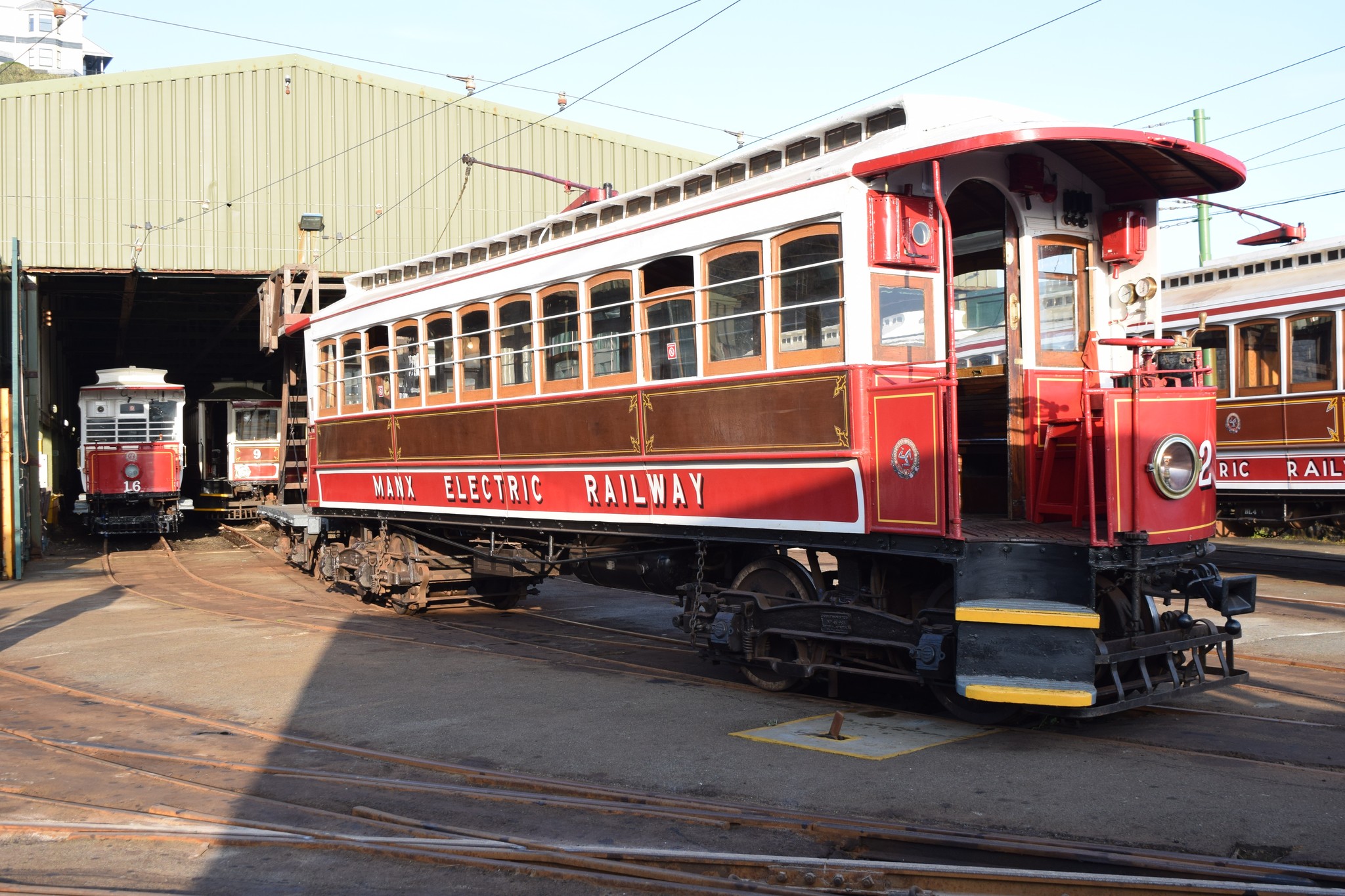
[72,364,196,541]
[182,377,291,522]
[291,87,1345,728]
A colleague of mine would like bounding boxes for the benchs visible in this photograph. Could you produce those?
[957,375,1008,514]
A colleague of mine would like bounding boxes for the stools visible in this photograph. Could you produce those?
[1032,417,1103,528]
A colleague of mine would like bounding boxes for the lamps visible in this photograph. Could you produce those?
[299,212,323,231]
